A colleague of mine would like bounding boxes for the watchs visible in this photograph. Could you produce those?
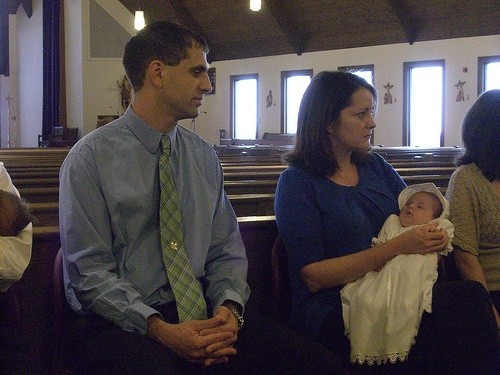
[227,306,244,331]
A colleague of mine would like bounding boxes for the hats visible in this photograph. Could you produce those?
[398,182,450,220]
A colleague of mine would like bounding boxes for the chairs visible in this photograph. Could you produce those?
[37,134,52,147]
[52,247,70,364]
[269,235,292,319]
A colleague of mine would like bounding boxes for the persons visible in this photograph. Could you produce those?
[58,19,342,375]
[274,70,500,375]
[339,182,455,366]
[0,162,34,294]
[445,89,500,331]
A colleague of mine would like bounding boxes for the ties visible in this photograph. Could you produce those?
[159,135,208,323]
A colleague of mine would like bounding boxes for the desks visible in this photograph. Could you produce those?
[0,144,466,340]
